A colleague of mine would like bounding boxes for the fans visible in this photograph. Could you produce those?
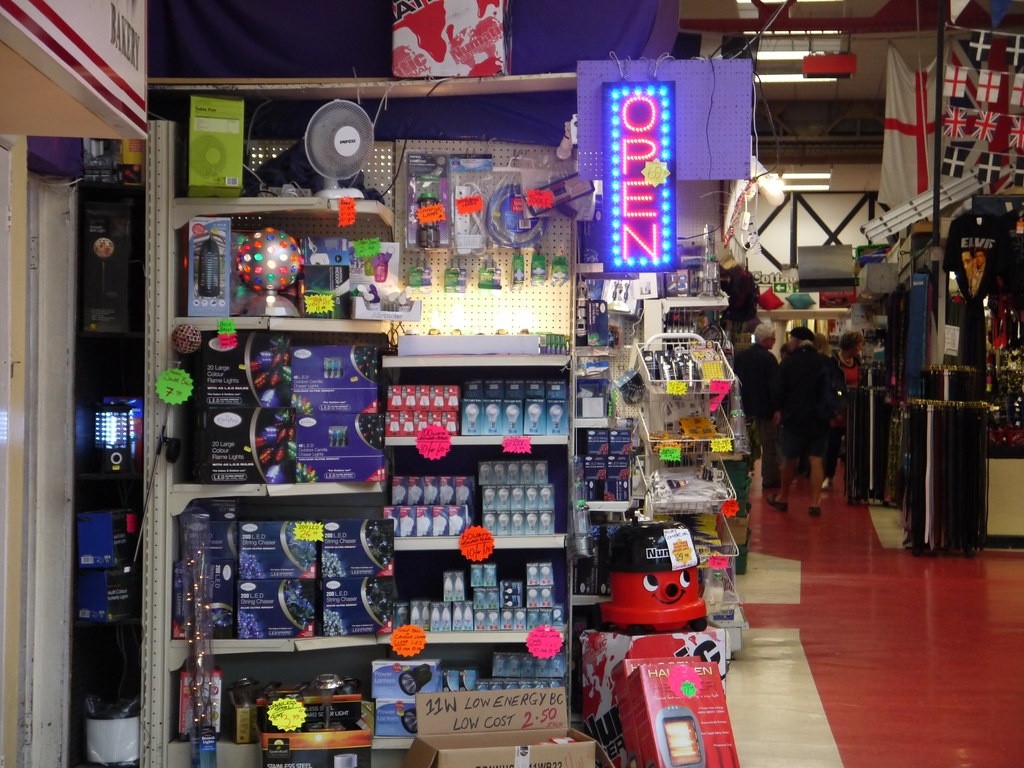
[303,99,375,198]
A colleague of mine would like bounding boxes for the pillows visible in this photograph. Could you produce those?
[785,294,816,310]
[757,286,784,311]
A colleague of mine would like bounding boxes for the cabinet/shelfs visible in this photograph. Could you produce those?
[158,120,635,768]
[22,120,156,767]
[636,333,740,653]
[574,256,750,659]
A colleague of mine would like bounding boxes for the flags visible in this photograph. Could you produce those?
[1011,74,1024,105]
[968,31,993,62]
[943,65,968,97]
[942,106,968,139]
[971,110,1000,142]
[1009,116,1024,148]
[1004,36,1024,66]
[977,152,1002,183]
[1014,156,1024,186]
[877,39,1024,217]
[976,69,1001,103]
[941,147,971,178]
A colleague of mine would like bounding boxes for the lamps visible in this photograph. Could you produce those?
[555,121,573,160]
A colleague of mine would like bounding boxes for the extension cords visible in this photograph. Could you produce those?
[268,186,311,198]
[454,185,471,254]
[654,466,712,511]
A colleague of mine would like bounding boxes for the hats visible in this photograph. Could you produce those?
[789,327,815,341]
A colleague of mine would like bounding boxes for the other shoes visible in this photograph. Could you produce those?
[808,506,821,516]
[762,480,782,489]
[822,477,830,489]
[767,494,788,512]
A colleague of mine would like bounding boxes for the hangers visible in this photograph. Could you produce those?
[968,198,990,216]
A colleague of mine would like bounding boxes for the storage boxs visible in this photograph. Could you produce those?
[172,500,396,640]
[383,461,555,538]
[104,396,143,472]
[392,0,513,78]
[372,652,567,736]
[81,138,145,188]
[186,331,388,484]
[633,269,693,300]
[525,172,595,216]
[402,687,617,768]
[180,665,222,743]
[188,92,245,197]
[386,378,570,437]
[574,263,604,272]
[393,562,566,632]
[612,656,740,768]
[585,300,608,346]
[575,430,631,501]
[82,201,146,334]
[184,217,422,322]
[580,624,725,768]
[77,508,143,622]
[229,682,374,768]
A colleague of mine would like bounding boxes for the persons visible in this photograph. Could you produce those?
[766,327,836,516]
[779,343,792,360]
[813,332,838,364]
[821,330,864,490]
[974,248,985,276]
[733,324,781,488]
